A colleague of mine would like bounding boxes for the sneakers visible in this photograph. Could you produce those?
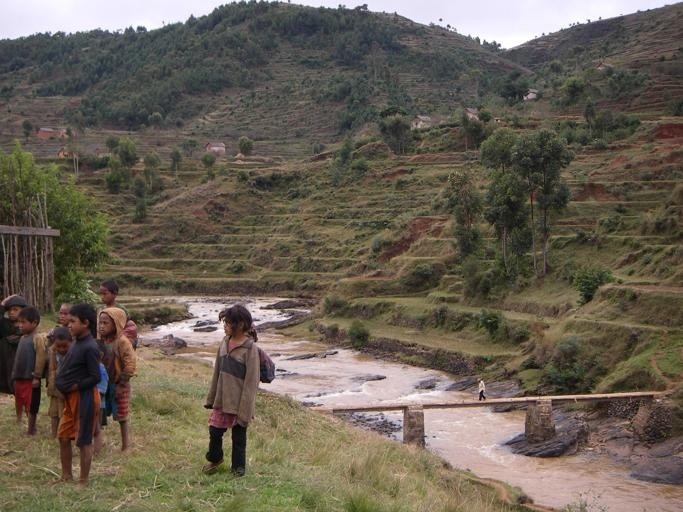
[205,458,224,471]
[229,469,245,480]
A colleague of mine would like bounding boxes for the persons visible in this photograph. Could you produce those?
[0,279,139,491]
[476,378,486,401]
[201,303,261,479]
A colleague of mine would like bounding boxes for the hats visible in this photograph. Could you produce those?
[4,296,27,309]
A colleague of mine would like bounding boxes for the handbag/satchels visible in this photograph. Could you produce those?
[257,346,275,383]
